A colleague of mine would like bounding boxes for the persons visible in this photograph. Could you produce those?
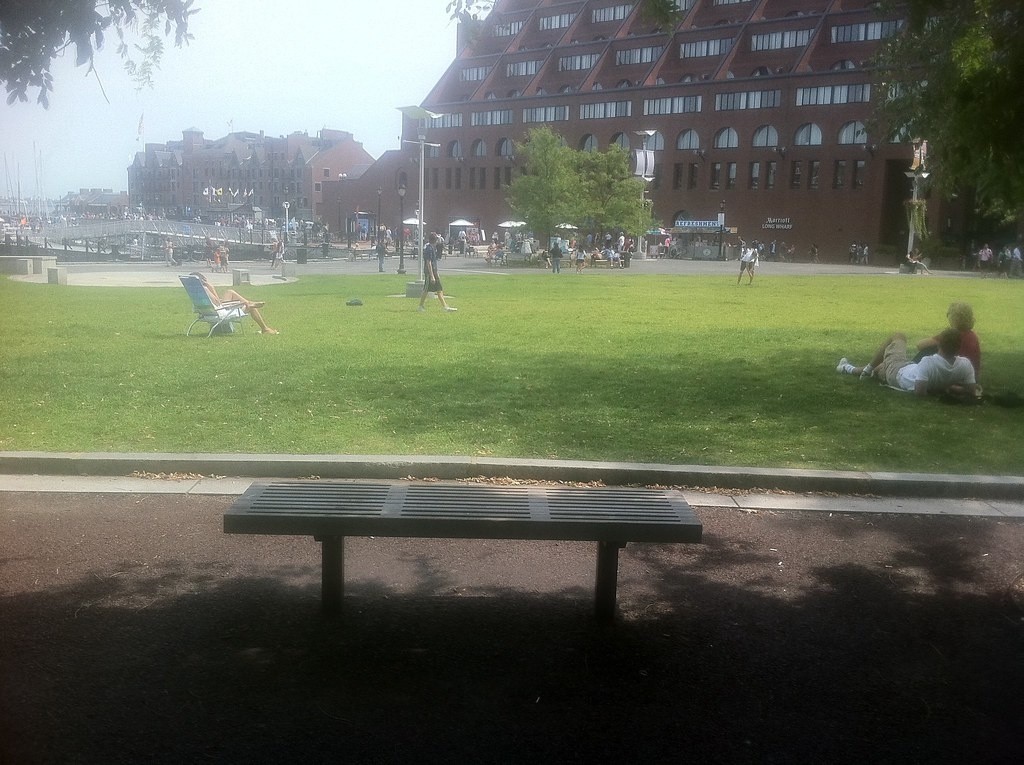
[911,303,981,377]
[418,232,458,312]
[848,241,869,264]
[542,232,631,273]
[204,234,229,274]
[483,229,533,265]
[836,328,976,396]
[978,243,1024,279]
[904,249,933,274]
[664,236,672,258]
[357,220,399,273]
[165,234,177,267]
[734,236,820,285]
[189,271,280,334]
[270,237,287,269]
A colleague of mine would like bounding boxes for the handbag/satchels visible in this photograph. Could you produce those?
[558,249,563,259]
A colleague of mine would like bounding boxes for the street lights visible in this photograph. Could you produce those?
[632,129,658,259]
[397,182,408,274]
[718,199,726,261]
[283,186,289,243]
[338,173,348,243]
[376,185,385,272]
[397,106,444,299]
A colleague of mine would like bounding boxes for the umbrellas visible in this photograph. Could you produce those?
[449,219,474,239]
[497,221,526,232]
[555,223,578,229]
[403,218,427,229]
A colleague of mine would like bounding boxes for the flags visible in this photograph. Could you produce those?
[203,187,254,196]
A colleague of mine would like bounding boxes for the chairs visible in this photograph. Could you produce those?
[179,275,244,338]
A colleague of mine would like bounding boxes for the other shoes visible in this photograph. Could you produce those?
[441,306,457,311]
[837,358,847,373]
[416,306,426,311]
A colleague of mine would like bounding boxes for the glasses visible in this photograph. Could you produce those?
[947,313,950,318]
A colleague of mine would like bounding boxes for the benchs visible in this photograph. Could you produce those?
[485,253,526,266]
[538,253,572,268]
[222,480,703,616]
[355,250,373,261]
[596,260,625,267]
